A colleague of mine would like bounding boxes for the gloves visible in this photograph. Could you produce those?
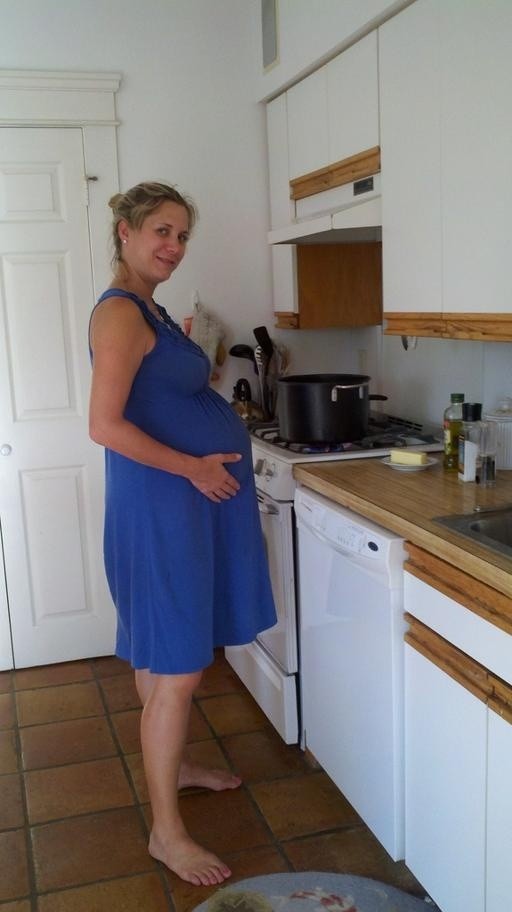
[184,303,225,381]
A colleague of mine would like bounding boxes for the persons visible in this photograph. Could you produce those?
[87,180,281,889]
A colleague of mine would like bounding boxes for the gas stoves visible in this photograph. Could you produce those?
[245,423,446,501]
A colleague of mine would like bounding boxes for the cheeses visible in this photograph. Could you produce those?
[391,447,428,466]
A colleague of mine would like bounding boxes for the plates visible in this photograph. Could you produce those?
[379,451,439,471]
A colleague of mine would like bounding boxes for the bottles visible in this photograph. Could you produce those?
[442,392,464,467]
[482,397,512,471]
[456,402,484,484]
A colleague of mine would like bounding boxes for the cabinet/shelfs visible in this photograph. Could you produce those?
[398,541,512,912]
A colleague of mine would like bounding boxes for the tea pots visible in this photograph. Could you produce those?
[229,378,265,427]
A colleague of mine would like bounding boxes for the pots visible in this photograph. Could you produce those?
[275,370,373,443]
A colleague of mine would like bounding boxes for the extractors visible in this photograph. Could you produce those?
[265,172,386,247]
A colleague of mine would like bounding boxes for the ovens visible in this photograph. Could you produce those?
[243,481,300,677]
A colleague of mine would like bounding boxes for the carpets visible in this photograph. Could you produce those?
[189,871,435,912]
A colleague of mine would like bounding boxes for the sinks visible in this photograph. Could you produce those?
[427,504,511,564]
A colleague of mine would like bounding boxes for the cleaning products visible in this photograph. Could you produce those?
[438,392,499,485]
